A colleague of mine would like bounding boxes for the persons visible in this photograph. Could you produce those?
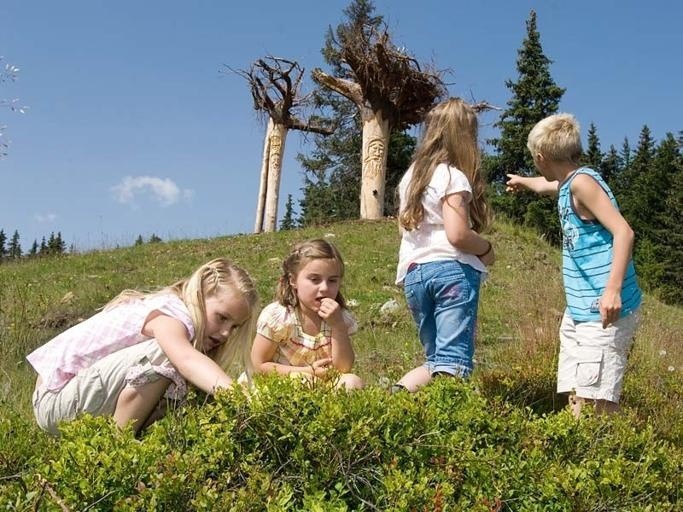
[23,258,258,446]
[506,113,646,415]
[236,241,364,395]
[395,97,497,391]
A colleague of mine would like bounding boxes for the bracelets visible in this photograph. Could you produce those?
[475,241,491,257]
